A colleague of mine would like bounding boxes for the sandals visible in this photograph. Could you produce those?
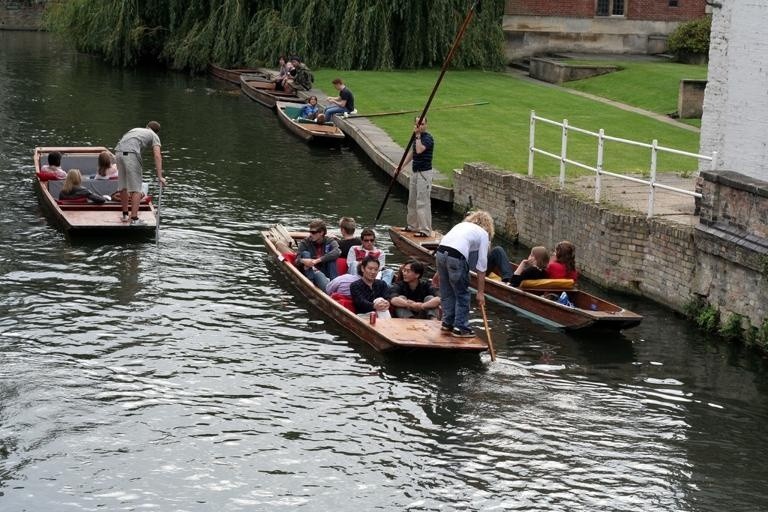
[399,225,418,232]
[414,230,432,238]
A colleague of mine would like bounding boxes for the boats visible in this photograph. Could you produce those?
[387,226,644,336]
[34,145,157,240]
[256,222,486,366]
[493,305,640,371]
[215,60,343,148]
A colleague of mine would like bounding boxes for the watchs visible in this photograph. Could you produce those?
[414,137,421,141]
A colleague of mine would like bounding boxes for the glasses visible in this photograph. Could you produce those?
[308,229,322,235]
[360,237,376,242]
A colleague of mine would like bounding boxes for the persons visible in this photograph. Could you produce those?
[113,120,167,228]
[281,62,295,89]
[485,243,548,296]
[276,216,363,258]
[94,150,118,180]
[349,254,392,319]
[394,114,434,239]
[270,56,285,91]
[285,55,314,91]
[346,228,395,287]
[58,168,112,204]
[547,238,578,287]
[293,216,342,282]
[314,113,326,125]
[393,254,416,283]
[299,94,318,118]
[389,258,441,321]
[324,78,355,121]
[431,210,496,339]
[41,150,67,179]
[325,262,364,296]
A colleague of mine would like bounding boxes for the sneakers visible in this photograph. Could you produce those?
[130,217,150,228]
[119,212,131,223]
[440,321,477,339]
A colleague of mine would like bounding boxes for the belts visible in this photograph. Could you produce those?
[436,246,466,262]
